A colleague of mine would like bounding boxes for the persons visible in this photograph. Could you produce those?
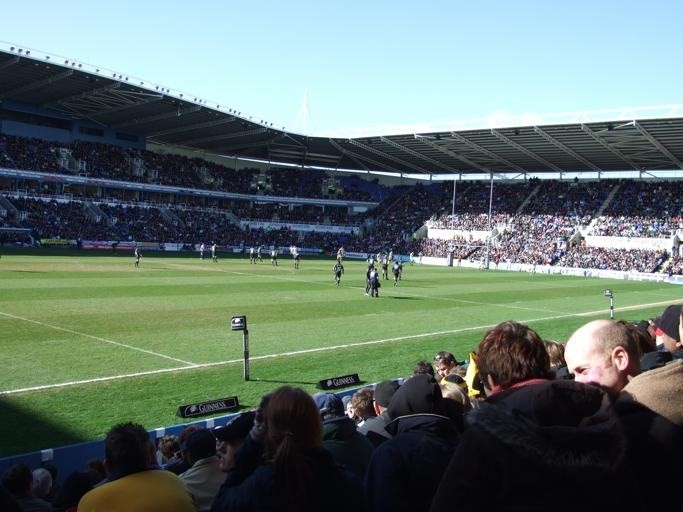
[364,265,373,296]
[381,255,388,281]
[2,131,392,269]
[397,262,402,283]
[391,262,401,287]
[332,260,344,287]
[1,304,682,512]
[369,268,379,298]
[328,176,683,276]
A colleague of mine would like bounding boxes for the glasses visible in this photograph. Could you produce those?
[433,356,449,362]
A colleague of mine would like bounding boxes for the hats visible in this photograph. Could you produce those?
[313,391,348,425]
[654,305,681,341]
[30,468,52,498]
[375,381,400,405]
[211,410,256,447]
